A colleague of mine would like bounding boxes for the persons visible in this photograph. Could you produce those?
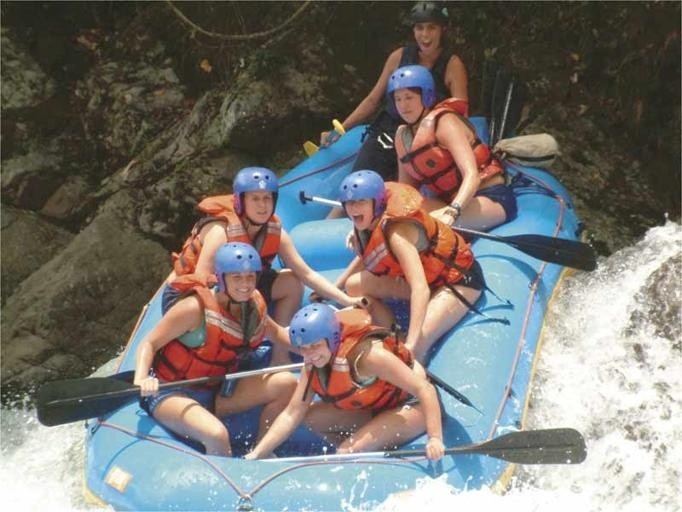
[135,239,303,457]
[307,170,486,363]
[241,299,446,460]
[343,65,518,250]
[318,1,469,147]
[174,167,369,365]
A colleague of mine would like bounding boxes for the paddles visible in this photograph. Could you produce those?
[298,191,596,271]
[35,362,304,428]
[490,71,527,165]
[256,428,585,465]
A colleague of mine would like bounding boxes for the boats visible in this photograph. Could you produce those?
[77,116,582,512]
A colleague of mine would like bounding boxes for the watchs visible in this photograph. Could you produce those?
[447,202,462,216]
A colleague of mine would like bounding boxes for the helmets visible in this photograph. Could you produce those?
[386,64,436,107]
[409,0,453,26]
[233,165,280,216]
[212,240,263,294]
[337,169,385,217]
[288,301,341,352]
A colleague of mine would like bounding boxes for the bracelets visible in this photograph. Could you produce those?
[444,210,459,221]
